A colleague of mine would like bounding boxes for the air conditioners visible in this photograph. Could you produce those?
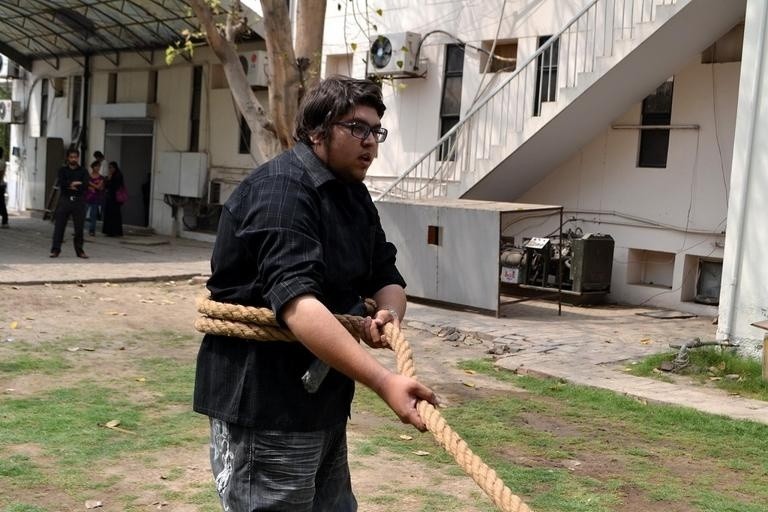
[0,100,22,124]
[367,31,422,76]
[1,53,19,79]
[236,51,270,90]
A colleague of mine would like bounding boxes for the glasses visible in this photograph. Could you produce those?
[333,122,387,142]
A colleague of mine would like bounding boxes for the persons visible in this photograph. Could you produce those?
[193,73,439,512]
[0,146,9,228]
[49,148,127,258]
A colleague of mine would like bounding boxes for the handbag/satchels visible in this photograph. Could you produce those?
[116,186,128,202]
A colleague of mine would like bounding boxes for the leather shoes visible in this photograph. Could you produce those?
[49,250,61,257]
[77,251,88,258]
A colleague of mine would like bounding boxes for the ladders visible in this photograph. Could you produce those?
[42,127,84,222]
